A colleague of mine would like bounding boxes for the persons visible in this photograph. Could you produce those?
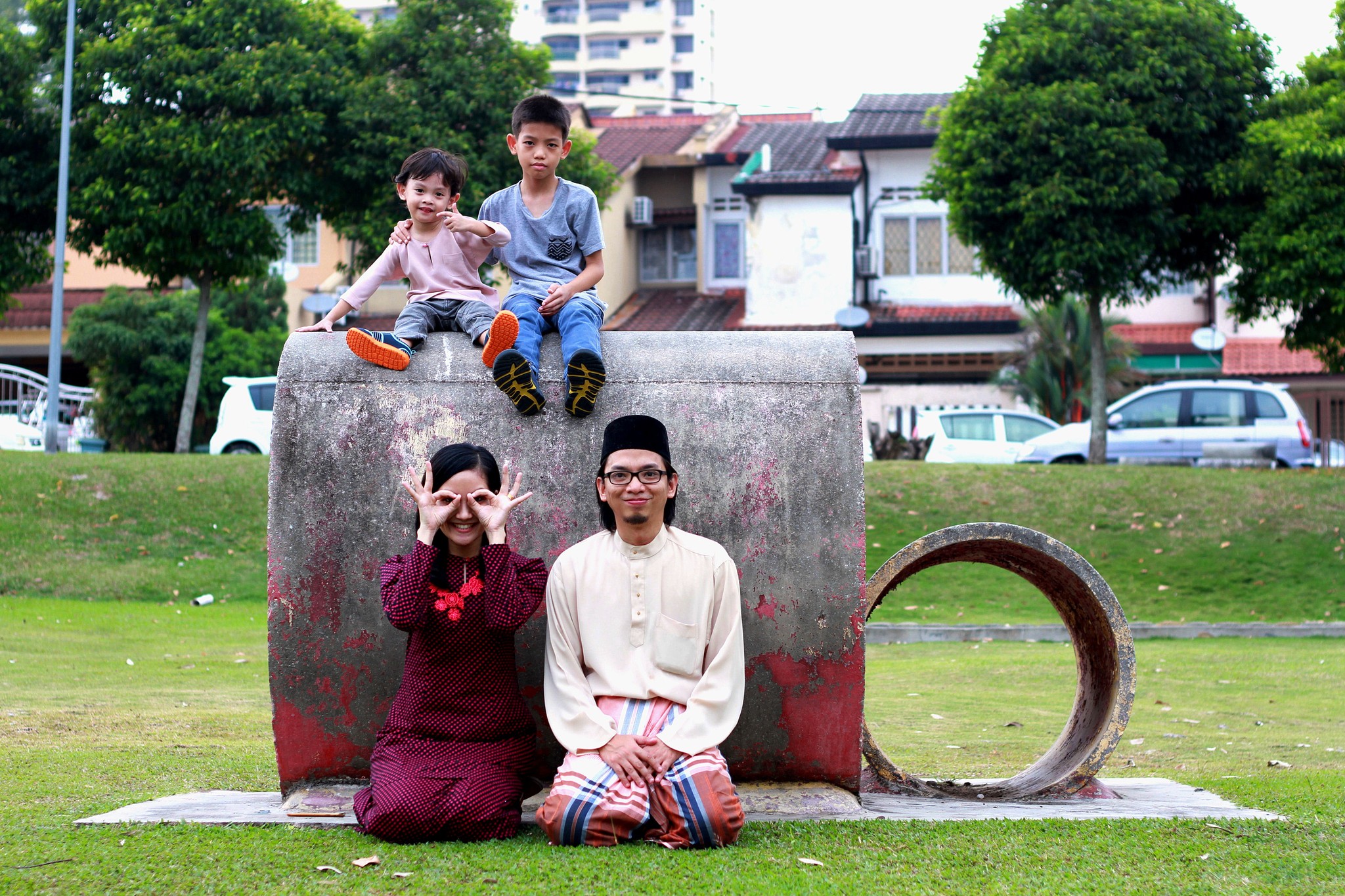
[354,442,548,841]
[388,95,608,419]
[294,148,519,367]
[536,414,745,852]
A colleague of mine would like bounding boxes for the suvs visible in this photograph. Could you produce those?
[912,404,1059,463]
[207,376,277,451]
[1013,376,1313,468]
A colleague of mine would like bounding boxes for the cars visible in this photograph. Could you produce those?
[0,396,98,453]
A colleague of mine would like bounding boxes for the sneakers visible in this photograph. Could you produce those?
[563,348,605,418]
[493,349,546,416]
[346,327,416,370]
[481,311,518,368]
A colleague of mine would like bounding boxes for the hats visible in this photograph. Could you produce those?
[600,415,671,468]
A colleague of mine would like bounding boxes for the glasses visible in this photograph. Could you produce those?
[604,469,668,485]
